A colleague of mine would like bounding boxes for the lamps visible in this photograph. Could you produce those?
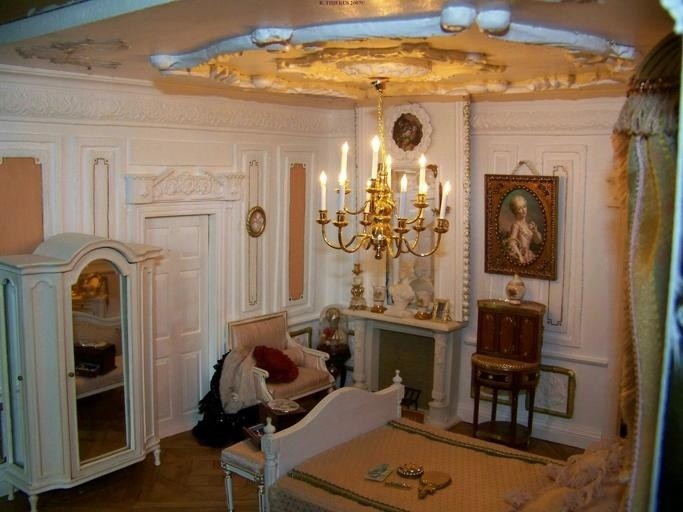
[315,76,451,261]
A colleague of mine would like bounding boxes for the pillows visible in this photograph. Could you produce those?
[282,347,303,366]
[252,345,298,382]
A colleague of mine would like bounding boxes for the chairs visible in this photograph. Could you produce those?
[227,311,337,431]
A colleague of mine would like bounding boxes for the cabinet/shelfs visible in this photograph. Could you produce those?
[1,231,166,512]
[471,299,545,447]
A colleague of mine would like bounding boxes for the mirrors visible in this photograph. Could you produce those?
[384,169,437,312]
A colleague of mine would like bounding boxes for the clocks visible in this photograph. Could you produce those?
[246,206,266,238]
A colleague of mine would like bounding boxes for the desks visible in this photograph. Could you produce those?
[319,344,350,388]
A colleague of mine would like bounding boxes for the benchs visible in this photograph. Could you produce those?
[220,436,269,512]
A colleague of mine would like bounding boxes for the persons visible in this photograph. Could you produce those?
[506,193,543,265]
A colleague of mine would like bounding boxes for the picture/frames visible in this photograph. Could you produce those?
[431,297,452,323]
[289,327,313,349]
[484,175,558,280]
[471,371,515,404]
[525,365,575,420]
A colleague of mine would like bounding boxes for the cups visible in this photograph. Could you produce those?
[370,285,387,313]
[414,289,432,319]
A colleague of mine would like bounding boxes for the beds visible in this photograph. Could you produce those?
[257,371,632,512]
